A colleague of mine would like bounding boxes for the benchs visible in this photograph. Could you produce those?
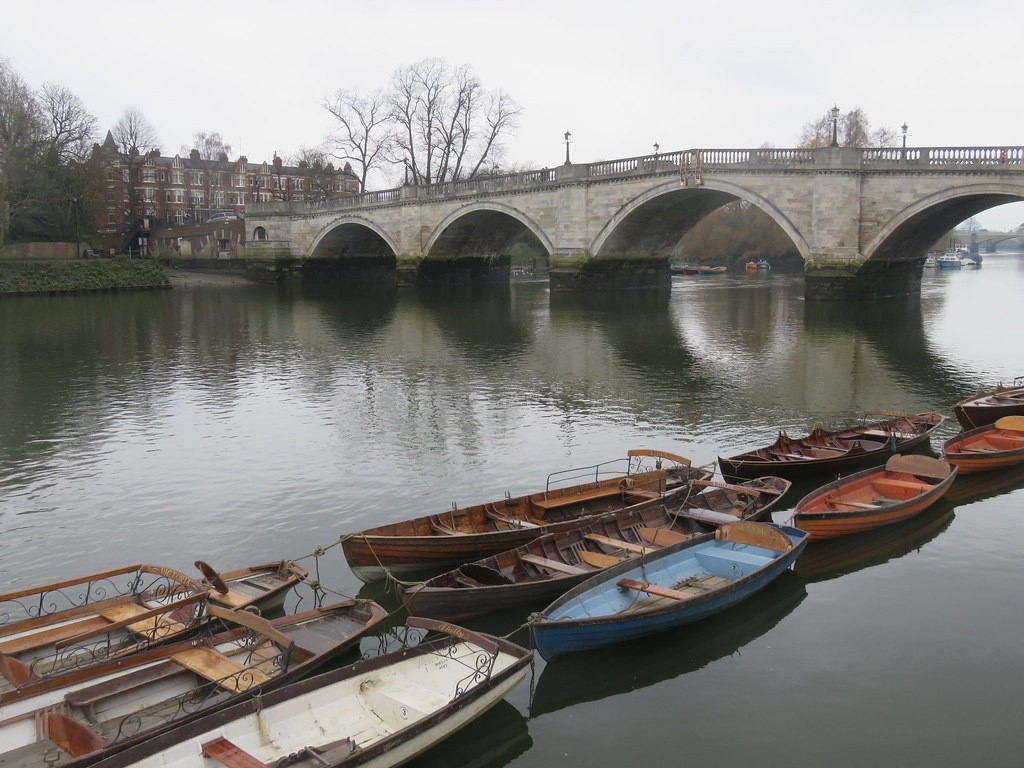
[364,678,451,727]
[975,401,1000,405]
[276,626,335,663]
[172,647,273,695]
[856,429,918,439]
[534,486,625,519]
[434,526,469,535]
[959,447,988,452]
[771,450,815,460]
[207,588,253,607]
[617,579,696,600]
[984,434,1024,443]
[625,489,660,503]
[457,575,487,588]
[993,396,1024,402]
[489,515,537,528]
[829,499,884,509]
[0,617,114,656]
[872,478,935,491]
[584,533,655,554]
[100,603,190,641]
[202,737,269,768]
[695,546,774,580]
[671,508,742,527]
[810,445,850,454]
[64,660,186,724]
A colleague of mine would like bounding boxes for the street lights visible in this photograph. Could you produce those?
[900,121,908,147]
[403,156,410,186]
[563,130,572,165]
[653,141,660,155]
[831,102,840,146]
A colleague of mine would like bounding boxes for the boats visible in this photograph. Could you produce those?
[954,376,1024,431]
[400,472,793,624]
[339,449,715,583]
[942,415,1024,475]
[0,597,391,768]
[925,252,977,268]
[683,264,728,275]
[717,409,946,484]
[0,558,310,710]
[82,616,534,768]
[791,453,960,544]
[530,521,811,661]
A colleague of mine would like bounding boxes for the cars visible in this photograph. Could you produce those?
[206,211,238,222]
